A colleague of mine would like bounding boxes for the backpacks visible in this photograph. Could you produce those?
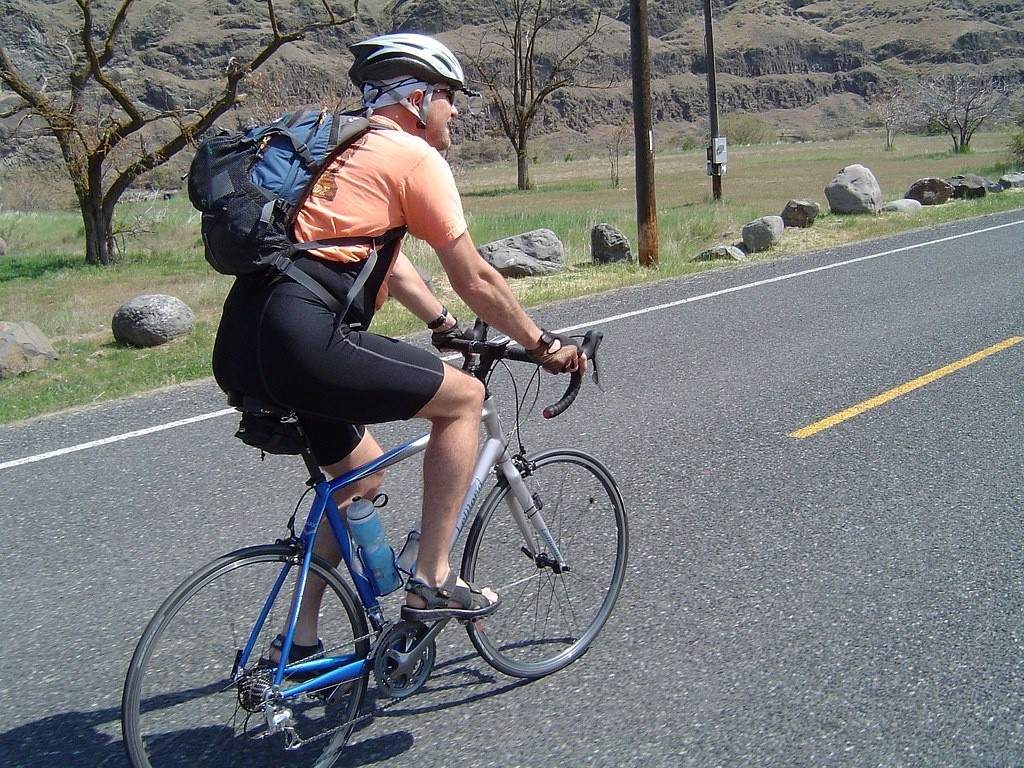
[188,106,400,276]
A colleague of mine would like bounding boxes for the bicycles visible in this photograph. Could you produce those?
[120,314,630,768]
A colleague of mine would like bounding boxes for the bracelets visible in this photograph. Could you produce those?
[428,305,448,329]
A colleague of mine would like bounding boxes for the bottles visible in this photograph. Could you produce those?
[347,493,398,595]
[399,509,421,576]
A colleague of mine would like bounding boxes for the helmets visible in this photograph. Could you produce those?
[348,32,465,93]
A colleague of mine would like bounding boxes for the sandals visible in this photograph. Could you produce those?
[257,634,349,695]
[400,569,502,621]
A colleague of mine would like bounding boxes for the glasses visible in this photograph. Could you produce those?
[420,88,455,107]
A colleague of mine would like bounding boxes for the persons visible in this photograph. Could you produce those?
[211,34,587,683]
